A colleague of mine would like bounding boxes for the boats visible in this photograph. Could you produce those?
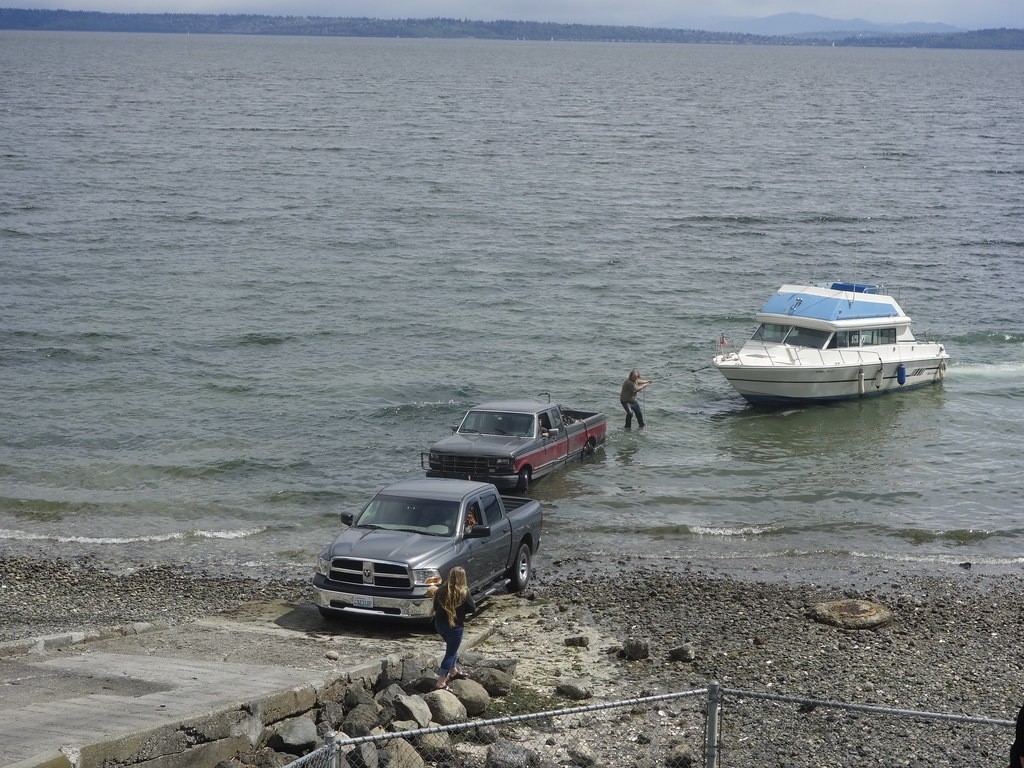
[712,275,951,406]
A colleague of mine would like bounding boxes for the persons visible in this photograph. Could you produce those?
[464,507,479,530]
[619,367,652,429]
[537,417,550,437]
[431,564,477,694]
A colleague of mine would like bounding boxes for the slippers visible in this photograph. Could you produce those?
[449,672,470,680]
[436,685,454,693]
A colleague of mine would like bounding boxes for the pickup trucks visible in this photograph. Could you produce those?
[309,477,543,629]
[420,399,607,494]
